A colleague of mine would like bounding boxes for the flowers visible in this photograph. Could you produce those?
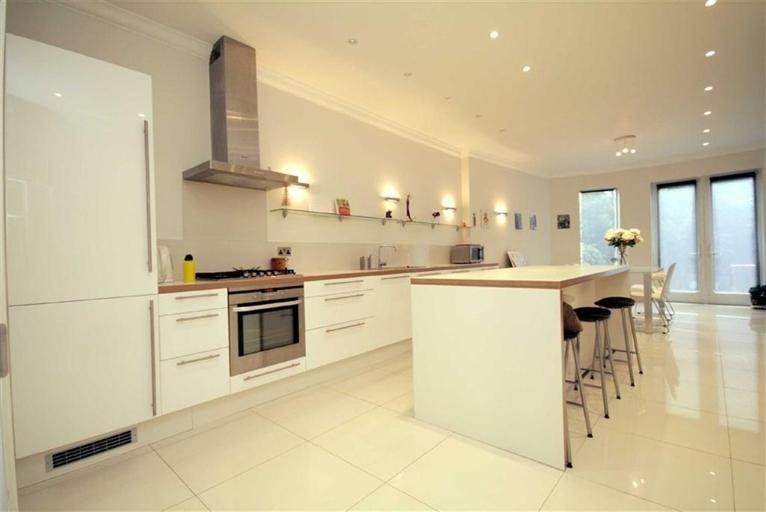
[603,225,644,265]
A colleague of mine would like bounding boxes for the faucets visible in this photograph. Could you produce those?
[376,244,398,268]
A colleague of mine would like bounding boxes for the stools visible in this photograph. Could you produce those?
[560,296,594,470]
[574,303,622,421]
[594,295,647,390]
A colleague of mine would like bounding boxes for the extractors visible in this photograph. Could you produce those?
[181,38,301,192]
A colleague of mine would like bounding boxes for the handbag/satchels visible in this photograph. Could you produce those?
[564,301,582,332]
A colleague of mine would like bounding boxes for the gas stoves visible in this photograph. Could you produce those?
[195,267,295,281]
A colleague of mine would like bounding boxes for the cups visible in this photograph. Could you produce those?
[360,256,370,268]
[271,257,286,270]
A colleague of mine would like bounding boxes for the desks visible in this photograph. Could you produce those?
[409,262,632,471]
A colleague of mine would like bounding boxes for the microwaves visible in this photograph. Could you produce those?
[450,243,484,264]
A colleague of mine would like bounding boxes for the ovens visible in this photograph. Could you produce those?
[228,286,305,376]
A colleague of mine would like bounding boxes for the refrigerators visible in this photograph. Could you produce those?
[0,33,160,460]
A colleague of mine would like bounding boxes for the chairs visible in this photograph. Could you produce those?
[631,261,678,334]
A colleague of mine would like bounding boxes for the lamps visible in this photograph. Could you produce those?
[611,133,640,159]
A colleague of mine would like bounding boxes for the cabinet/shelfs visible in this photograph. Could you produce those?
[376,271,412,350]
[158,284,231,417]
[305,275,376,372]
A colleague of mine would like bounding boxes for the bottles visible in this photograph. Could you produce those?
[183,254,196,281]
[157,247,175,284]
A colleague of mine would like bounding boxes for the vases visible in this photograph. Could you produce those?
[611,247,630,265]
